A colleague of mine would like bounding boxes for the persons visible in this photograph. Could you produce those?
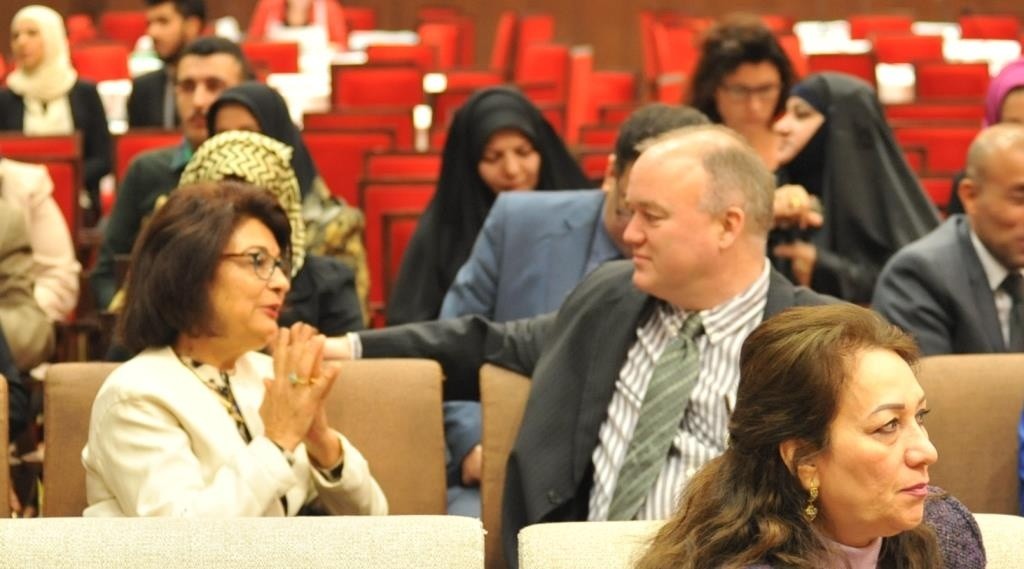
[0,326,43,519]
[78,177,391,520]
[387,82,603,401]
[444,97,717,518]
[946,58,1024,218]
[1,196,54,372]
[105,127,368,364]
[107,81,374,327]
[631,302,987,569]
[312,120,867,568]
[91,31,249,318]
[2,4,113,224]
[770,71,950,309]
[125,0,209,136]
[684,18,816,189]
[867,119,1024,360]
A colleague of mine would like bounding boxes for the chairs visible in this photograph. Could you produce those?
[2,1,1022,568]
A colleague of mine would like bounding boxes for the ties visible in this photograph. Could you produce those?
[606,313,705,521]
[999,271,1024,353]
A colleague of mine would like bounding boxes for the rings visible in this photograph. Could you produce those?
[309,376,318,384]
[284,369,307,387]
[791,195,801,207]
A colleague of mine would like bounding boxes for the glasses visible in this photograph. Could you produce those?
[718,79,786,107]
[614,168,630,227]
[222,251,293,281]
